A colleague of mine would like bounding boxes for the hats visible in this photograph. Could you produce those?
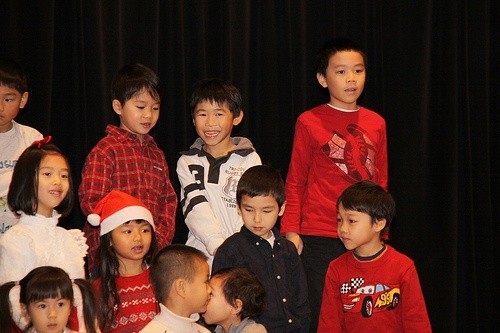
[87,190,155,236]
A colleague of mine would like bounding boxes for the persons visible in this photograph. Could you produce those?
[87,186,161,333]
[279,41,389,301]
[0,135,89,281]
[317,181,432,333]
[176,78,262,273]
[139,244,212,333]
[0,266,96,333]
[0,70,45,235]
[202,265,268,333]
[78,62,173,276]
[211,165,312,333]
